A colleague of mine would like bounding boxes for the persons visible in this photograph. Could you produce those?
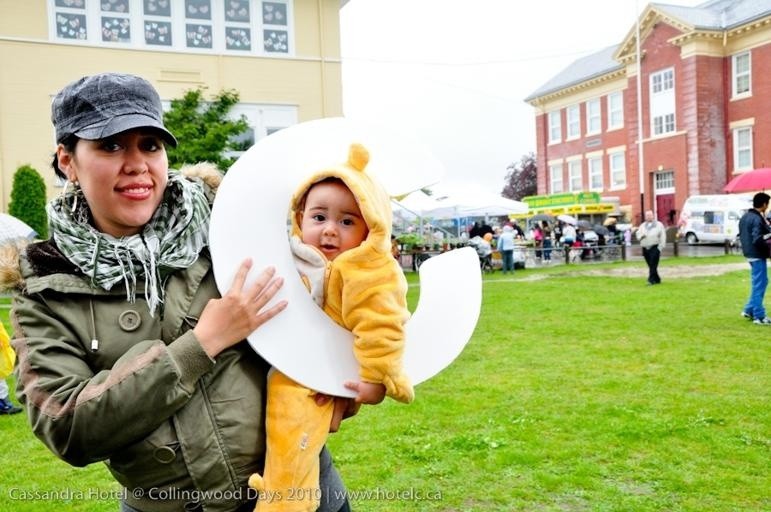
[392,214,634,276]
[637,209,667,285]
[738,191,771,325]
[248,142,416,511]
[0,71,365,511]
[0,320,24,416]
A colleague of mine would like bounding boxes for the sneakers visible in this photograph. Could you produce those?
[742,312,770,325]
[0,398,23,415]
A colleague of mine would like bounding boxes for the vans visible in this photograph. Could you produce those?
[677,193,754,245]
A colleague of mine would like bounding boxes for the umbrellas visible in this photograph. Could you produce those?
[720,160,771,193]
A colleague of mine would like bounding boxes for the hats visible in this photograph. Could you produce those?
[49,71,179,150]
